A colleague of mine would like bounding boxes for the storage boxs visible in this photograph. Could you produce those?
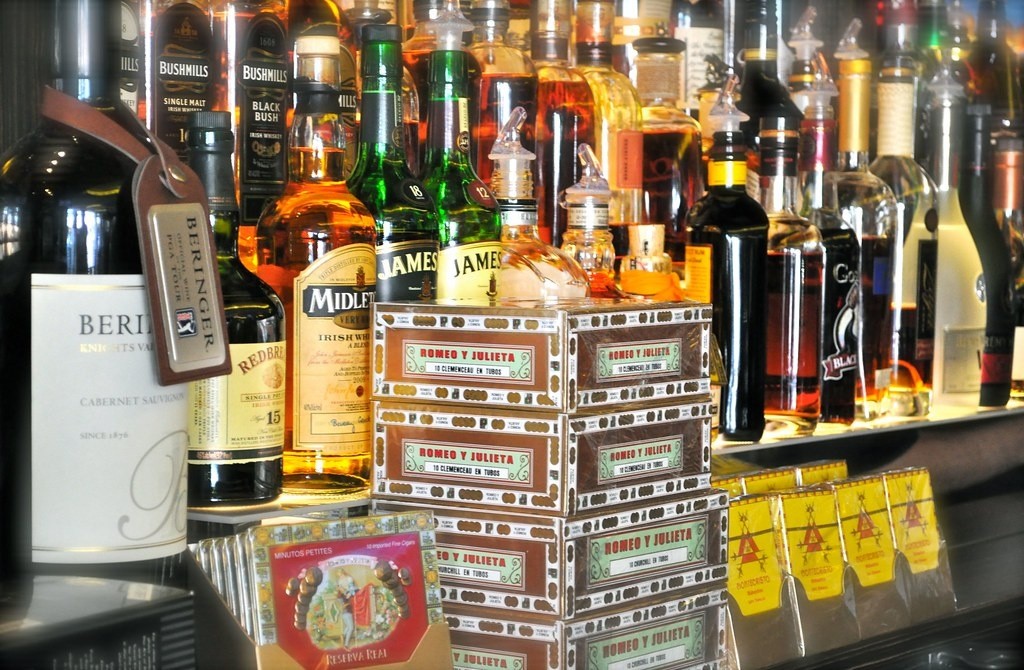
[369,300,957,670]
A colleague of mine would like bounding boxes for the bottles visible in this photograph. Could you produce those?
[0,0,1024,589]
